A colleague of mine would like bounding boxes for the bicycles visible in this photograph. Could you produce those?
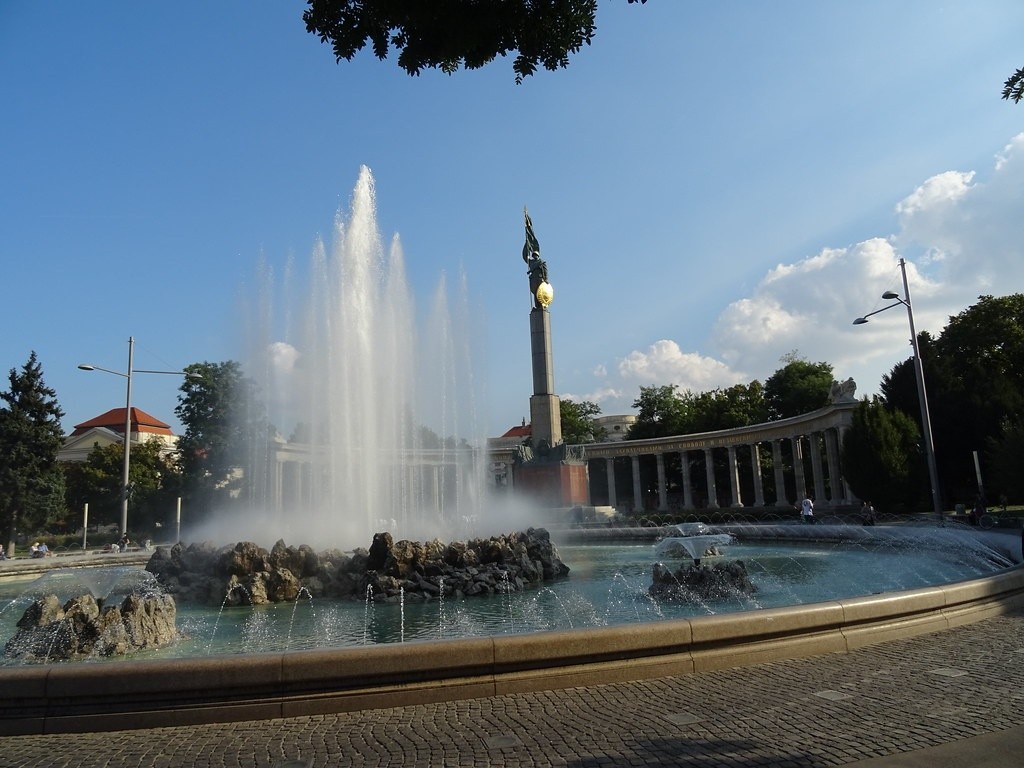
[978,505,1018,531]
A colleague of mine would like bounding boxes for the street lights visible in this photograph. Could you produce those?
[78,363,202,545]
[852,290,944,528]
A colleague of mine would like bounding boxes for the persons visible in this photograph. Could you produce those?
[526,251,548,309]
[109,543,120,553]
[859,498,875,526]
[800,494,814,524]
[117,533,130,553]
[29,541,50,559]
[0,544,6,561]
[144,537,151,551]
